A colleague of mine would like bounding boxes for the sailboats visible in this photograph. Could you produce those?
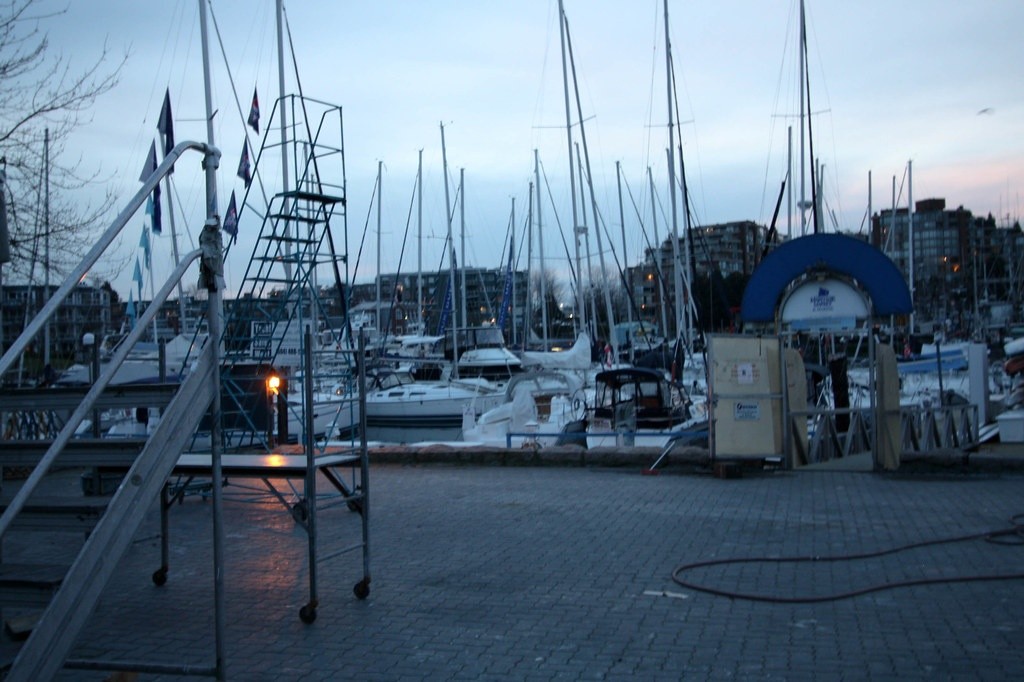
[19,0,1024,450]
